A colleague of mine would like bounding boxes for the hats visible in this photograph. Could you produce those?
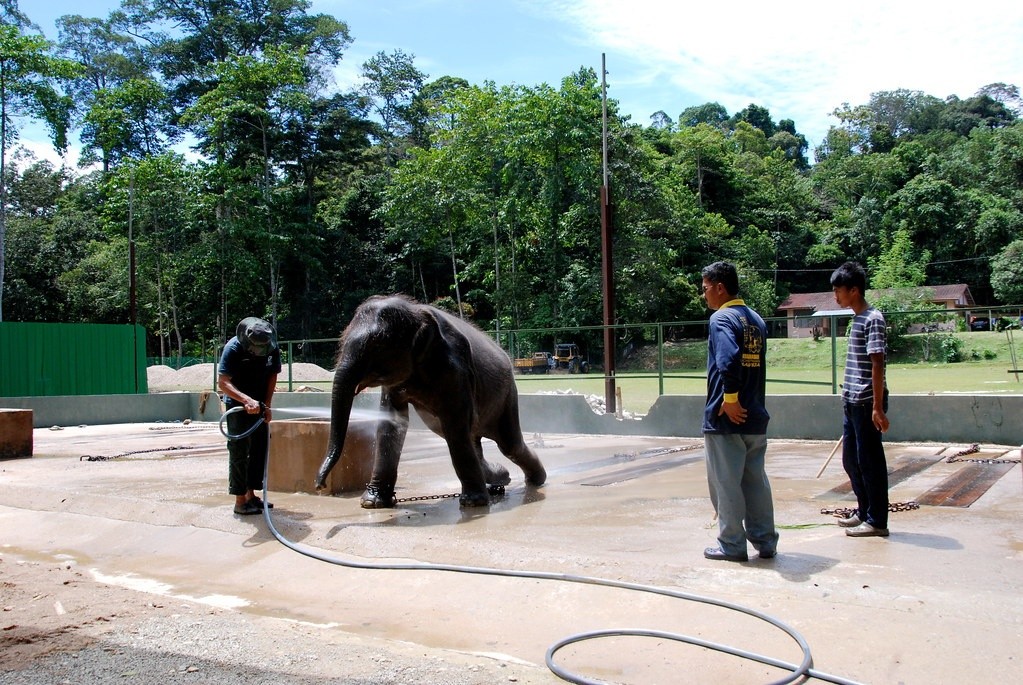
[236,316,277,357]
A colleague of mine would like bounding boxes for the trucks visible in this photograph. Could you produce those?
[514,352,555,375]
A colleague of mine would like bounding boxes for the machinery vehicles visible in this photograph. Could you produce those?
[552,344,589,374]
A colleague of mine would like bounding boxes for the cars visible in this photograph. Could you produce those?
[971,317,995,331]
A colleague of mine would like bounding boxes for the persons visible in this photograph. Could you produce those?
[547,354,555,369]
[700,260,779,562]
[830,260,891,536]
[218,316,282,515]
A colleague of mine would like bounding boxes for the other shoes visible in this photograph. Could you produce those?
[758,551,777,559]
[845,521,890,537]
[246,496,273,508]
[234,502,262,515]
[704,546,748,562]
[838,514,862,527]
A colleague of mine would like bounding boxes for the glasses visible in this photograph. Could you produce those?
[702,283,718,292]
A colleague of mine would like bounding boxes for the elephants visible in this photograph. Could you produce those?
[313,290,547,510]
